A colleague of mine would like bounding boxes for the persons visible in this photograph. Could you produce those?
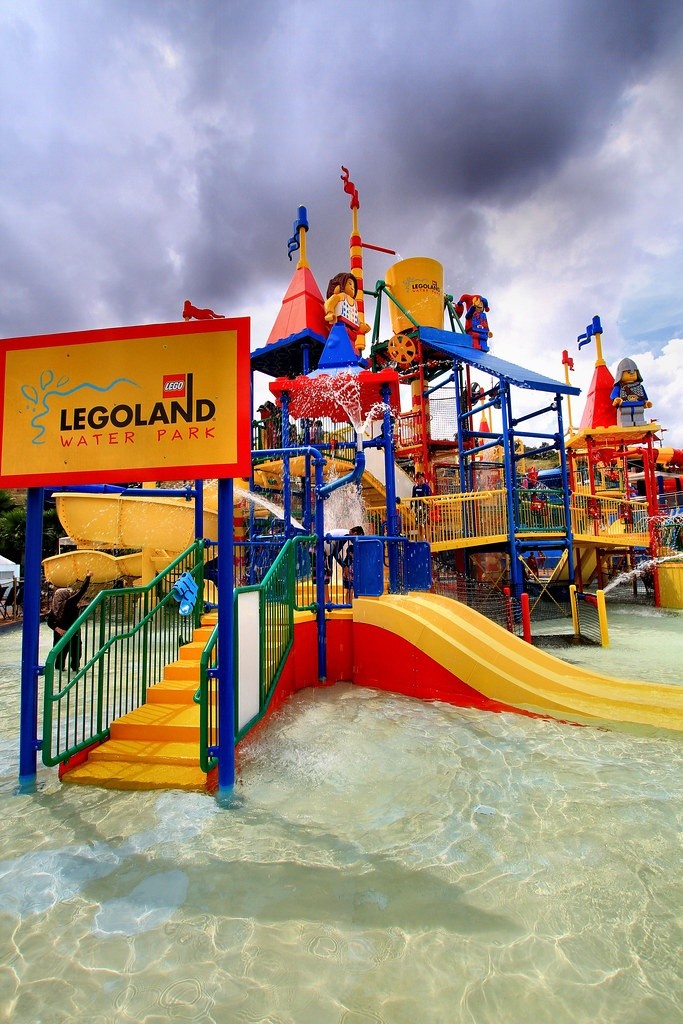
[531,492,548,501]
[453,293,494,352]
[312,418,325,444]
[528,549,547,579]
[272,410,284,448]
[608,358,653,427]
[410,472,432,544]
[318,272,371,371]
[308,526,364,603]
[46,570,93,672]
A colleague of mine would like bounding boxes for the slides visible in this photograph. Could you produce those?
[352,594,682,736]
[545,520,624,590]
[40,468,255,607]
